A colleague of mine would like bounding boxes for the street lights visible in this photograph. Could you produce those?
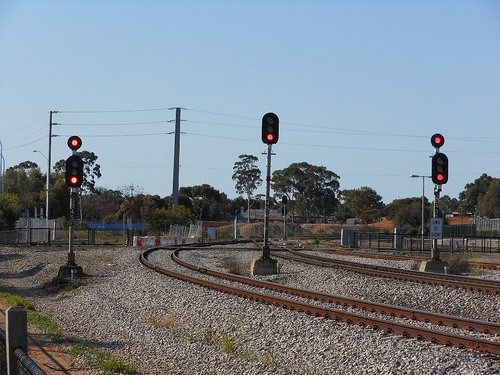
[32,149,50,221]
[410,174,425,252]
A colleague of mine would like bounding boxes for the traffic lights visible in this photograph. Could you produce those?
[261,112,280,144]
[67,136,82,151]
[65,155,83,188]
[430,133,445,149]
[431,153,449,185]
